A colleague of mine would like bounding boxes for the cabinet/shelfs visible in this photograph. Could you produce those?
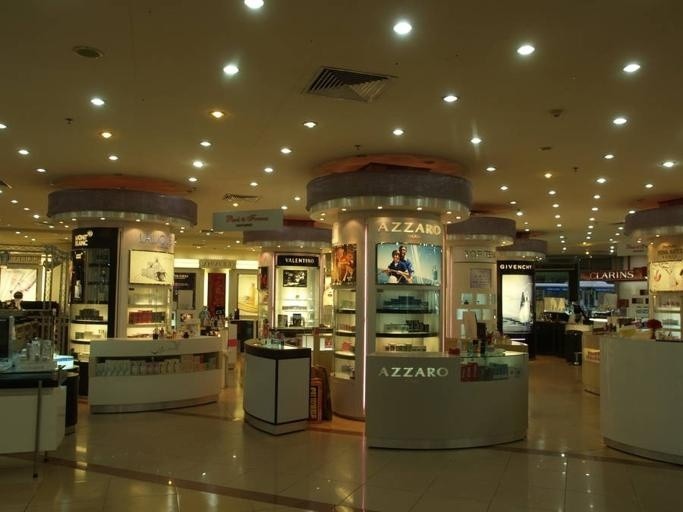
[55,246,174,396]
[329,284,445,422]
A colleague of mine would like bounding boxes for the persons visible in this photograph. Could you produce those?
[384,246,414,283]
[568,307,585,326]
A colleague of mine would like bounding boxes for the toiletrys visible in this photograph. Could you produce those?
[467,331,508,354]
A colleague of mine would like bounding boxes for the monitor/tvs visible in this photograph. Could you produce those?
[0,314,14,358]
